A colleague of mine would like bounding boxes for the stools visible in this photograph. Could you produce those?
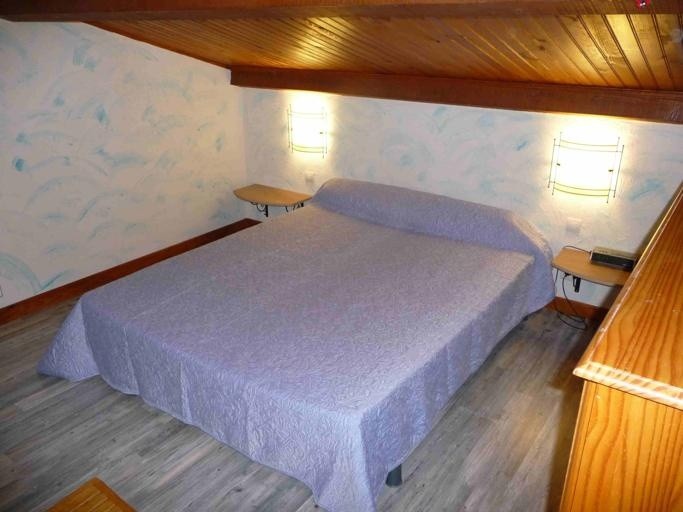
[47,477,137,512]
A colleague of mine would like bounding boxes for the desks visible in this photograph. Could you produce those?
[233,184,313,217]
[552,248,633,288]
[557,181,683,512]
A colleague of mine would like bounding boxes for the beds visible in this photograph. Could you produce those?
[36,177,555,512]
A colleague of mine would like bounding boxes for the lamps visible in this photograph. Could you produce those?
[548,132,625,203]
[286,103,327,158]
